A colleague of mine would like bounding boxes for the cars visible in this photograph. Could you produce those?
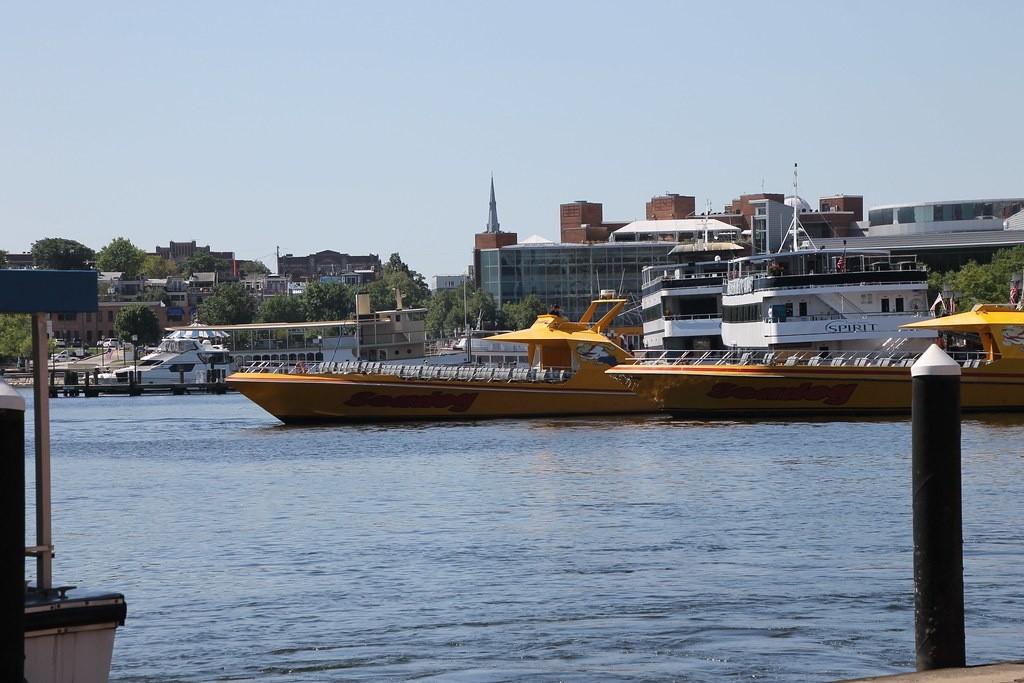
[52,354,80,363]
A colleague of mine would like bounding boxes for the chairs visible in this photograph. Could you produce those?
[733,352,981,368]
[317,362,566,384]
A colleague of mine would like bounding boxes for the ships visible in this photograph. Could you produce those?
[87,288,661,430]
[605,271,1024,416]
[640,162,939,362]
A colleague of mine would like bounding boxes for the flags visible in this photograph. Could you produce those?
[837,257,847,268]
[930,295,941,317]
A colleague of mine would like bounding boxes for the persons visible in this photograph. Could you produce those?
[663,306,674,321]
[133,345,138,361]
[546,304,559,317]
[106,347,112,359]
[768,260,781,276]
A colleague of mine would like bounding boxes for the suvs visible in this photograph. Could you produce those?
[102,337,118,349]
[68,337,83,349]
[52,338,66,347]
